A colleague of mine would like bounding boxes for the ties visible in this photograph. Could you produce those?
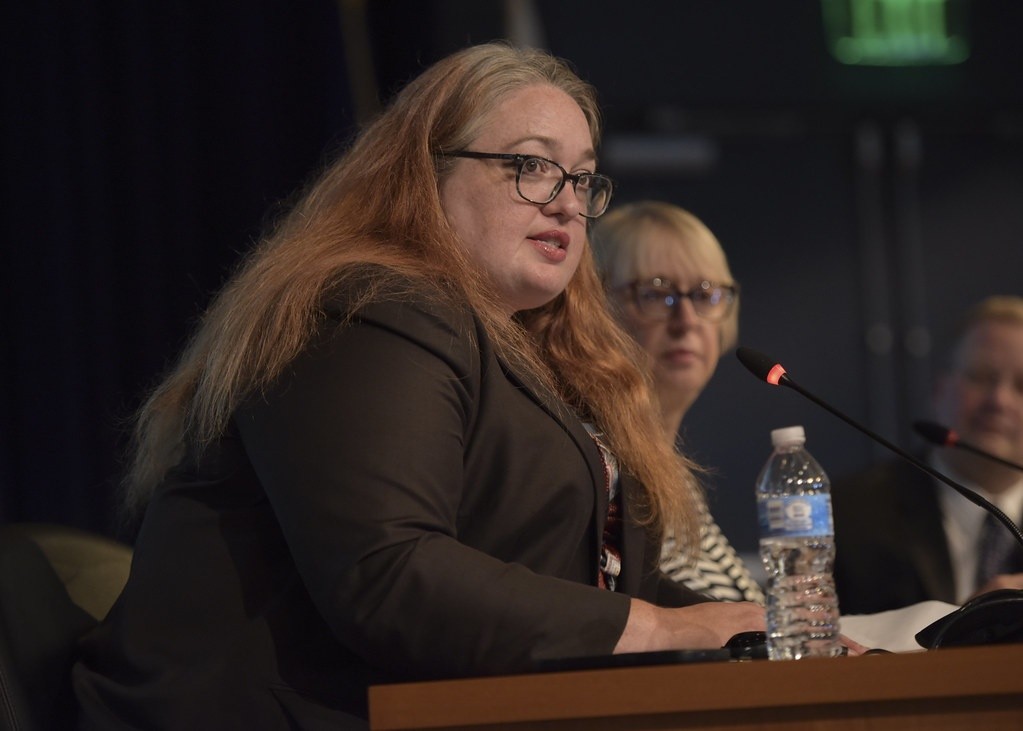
[975,510,1015,596]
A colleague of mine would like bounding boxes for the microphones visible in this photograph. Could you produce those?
[737,346,1023,649]
[913,418,1023,474]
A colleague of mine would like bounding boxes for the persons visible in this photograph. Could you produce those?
[828,296,1023,618]
[589,200,895,655]
[68,42,867,731]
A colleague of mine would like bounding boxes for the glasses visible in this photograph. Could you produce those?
[607,277,737,321]
[435,150,613,219]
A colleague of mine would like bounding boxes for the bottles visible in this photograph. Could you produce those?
[755,425,841,659]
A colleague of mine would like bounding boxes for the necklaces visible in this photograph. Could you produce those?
[586,432,623,577]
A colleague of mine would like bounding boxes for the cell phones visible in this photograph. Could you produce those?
[524,647,731,673]
